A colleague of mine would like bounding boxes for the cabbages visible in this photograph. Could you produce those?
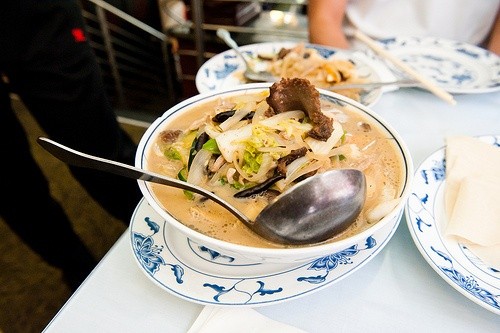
[164,91,403,223]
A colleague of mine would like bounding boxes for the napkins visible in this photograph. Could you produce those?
[186,306,305,333]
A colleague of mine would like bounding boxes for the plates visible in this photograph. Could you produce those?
[362,36,500,94]
[195,42,382,109]
[129,196,404,306]
[404,135,500,316]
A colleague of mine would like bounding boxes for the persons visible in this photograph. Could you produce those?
[307,1,500,55]
[0,0,145,290]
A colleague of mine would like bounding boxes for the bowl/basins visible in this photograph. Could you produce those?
[135,83,413,260]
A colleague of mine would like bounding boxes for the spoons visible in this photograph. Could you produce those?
[217,28,274,82]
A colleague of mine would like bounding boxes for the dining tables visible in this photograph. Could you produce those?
[43,86,500,333]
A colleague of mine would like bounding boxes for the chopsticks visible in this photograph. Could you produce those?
[353,30,454,103]
